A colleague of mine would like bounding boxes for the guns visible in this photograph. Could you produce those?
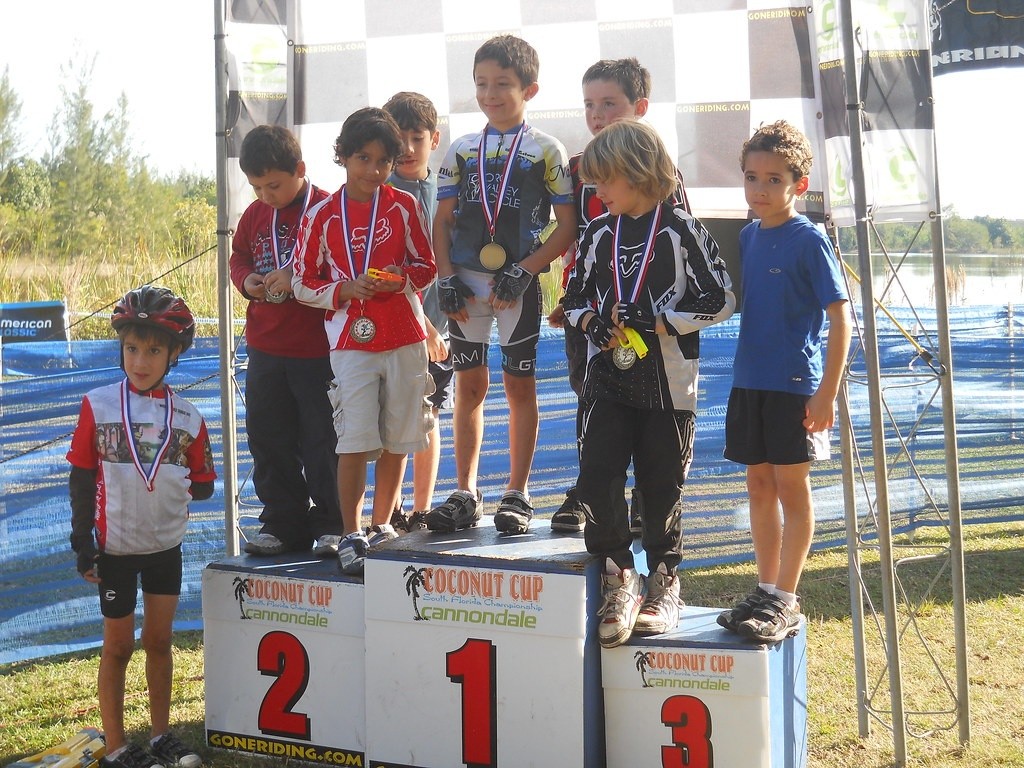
[368,268,407,283]
[617,325,648,360]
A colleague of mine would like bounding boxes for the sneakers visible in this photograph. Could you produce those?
[99,744,162,768]
[366,523,399,548]
[407,510,428,530]
[736,593,802,642]
[493,484,534,532]
[426,488,484,530]
[595,556,649,648]
[716,587,779,634]
[550,485,588,531]
[315,534,343,556]
[630,488,644,532]
[338,529,369,574]
[243,524,290,554]
[148,733,201,768]
[391,506,409,537]
[635,562,686,635]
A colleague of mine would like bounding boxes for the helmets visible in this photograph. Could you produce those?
[111,285,195,353]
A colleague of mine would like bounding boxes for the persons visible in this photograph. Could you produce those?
[290,92,452,570]
[717,121,852,641]
[550,57,691,538]
[561,122,735,650]
[67,288,216,768]
[420,35,577,531]
[230,121,339,558]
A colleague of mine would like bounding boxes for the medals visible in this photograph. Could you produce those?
[349,316,376,344]
[613,343,636,370]
[479,243,506,271]
[265,287,287,304]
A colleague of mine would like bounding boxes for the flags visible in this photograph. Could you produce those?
[218,3,1024,229]
